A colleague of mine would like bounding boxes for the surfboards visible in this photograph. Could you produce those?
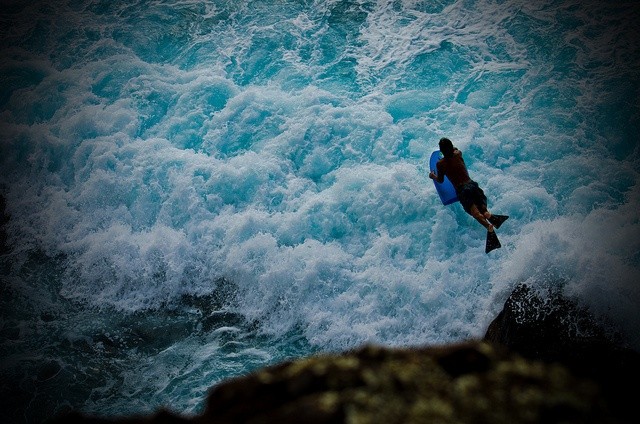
[430,150,461,206]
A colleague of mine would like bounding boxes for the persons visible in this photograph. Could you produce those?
[429,138,507,234]
